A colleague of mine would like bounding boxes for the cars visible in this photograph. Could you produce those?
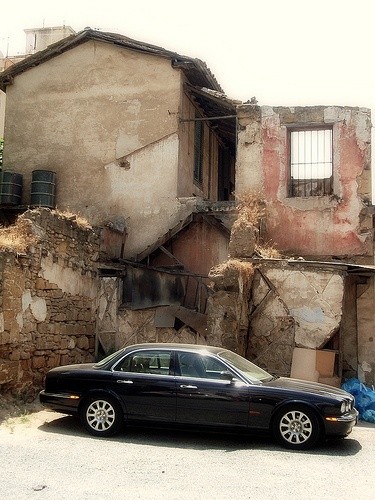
[38,342,359,450]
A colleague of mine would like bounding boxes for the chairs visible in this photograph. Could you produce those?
[181,355,199,377]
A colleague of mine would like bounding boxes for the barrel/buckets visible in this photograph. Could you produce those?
[0,172,22,205]
[31,171,56,207]
